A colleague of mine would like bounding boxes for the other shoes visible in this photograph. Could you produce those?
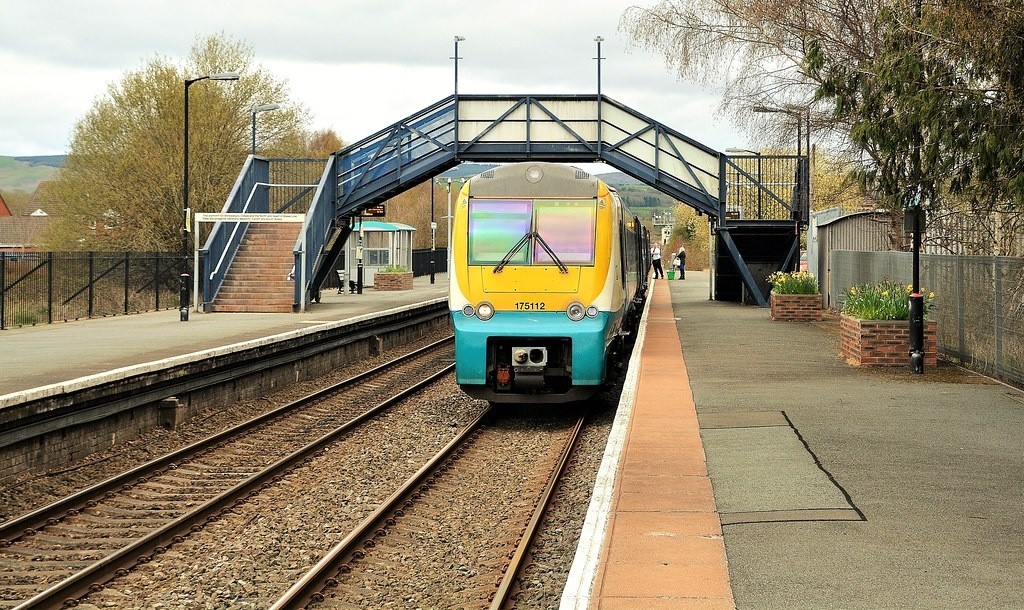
[653,277,658,279]
[678,277,685,280]
[661,276,663,279]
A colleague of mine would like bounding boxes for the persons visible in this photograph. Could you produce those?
[650,243,663,279]
[677,246,686,280]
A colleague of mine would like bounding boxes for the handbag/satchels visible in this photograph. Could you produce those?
[673,257,681,266]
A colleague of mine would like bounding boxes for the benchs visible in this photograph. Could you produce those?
[336,270,357,294]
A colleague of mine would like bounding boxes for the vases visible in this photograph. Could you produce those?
[770,290,823,322]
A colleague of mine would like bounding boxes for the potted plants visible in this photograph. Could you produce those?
[840,313,938,368]
[374,265,414,290]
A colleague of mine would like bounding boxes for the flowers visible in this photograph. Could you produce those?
[766,270,819,294]
[838,277,935,322]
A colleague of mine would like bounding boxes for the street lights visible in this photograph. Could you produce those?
[784,103,810,157]
[725,147,761,219]
[181,73,239,323]
[594,36,604,155]
[737,171,765,205]
[753,105,801,274]
[454,36,465,155]
[252,103,280,153]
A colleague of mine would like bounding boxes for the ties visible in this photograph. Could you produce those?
[652,248,655,257]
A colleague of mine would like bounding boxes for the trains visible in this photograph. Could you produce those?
[448,161,654,405]
